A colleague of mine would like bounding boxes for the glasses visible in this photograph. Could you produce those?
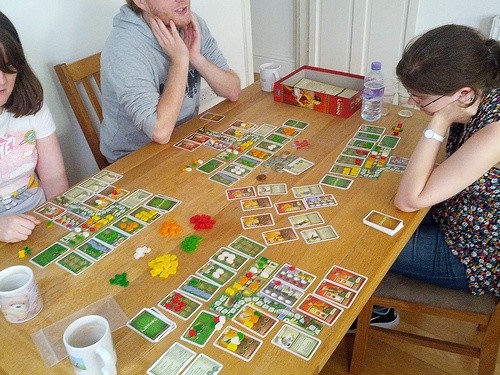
[407,92,446,110]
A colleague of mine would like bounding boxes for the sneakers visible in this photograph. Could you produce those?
[345,304,401,332]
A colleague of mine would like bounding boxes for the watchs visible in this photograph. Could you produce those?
[423,128,444,142]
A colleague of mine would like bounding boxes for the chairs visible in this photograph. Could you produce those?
[52,51,111,171]
[349,273,500,375]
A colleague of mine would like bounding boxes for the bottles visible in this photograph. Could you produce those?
[360,62,385,122]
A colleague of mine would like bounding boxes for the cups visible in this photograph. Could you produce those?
[259,63,281,93]
[0,265,43,325]
[62,315,117,375]
[397,89,415,119]
[381,87,396,117]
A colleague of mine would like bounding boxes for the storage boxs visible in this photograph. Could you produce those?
[274,65,365,118]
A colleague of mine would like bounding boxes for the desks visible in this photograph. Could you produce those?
[0,78,449,375]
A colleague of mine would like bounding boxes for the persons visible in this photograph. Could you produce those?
[350,25,500,332]
[100,1,240,164]
[0,9,68,242]
